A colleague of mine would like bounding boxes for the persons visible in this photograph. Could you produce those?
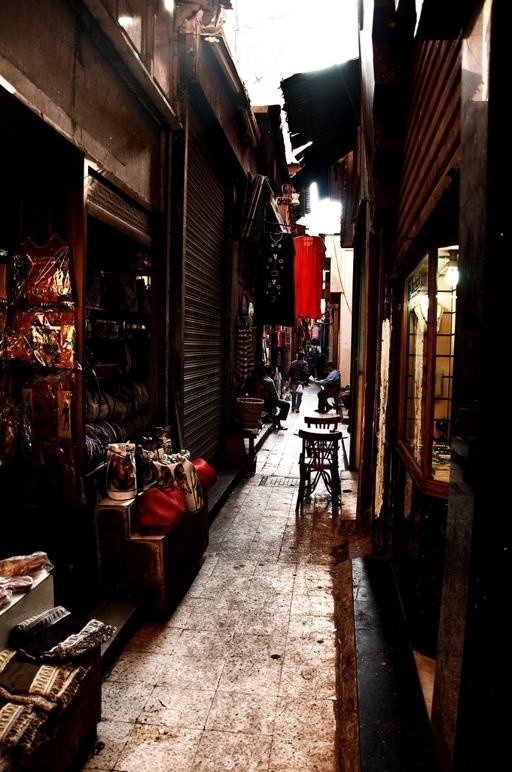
[308,345,320,381]
[261,364,291,431]
[313,359,341,413]
[283,352,309,413]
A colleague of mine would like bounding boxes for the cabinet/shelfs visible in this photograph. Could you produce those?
[94,433,181,602]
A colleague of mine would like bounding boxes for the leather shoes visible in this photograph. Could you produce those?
[315,405,332,413]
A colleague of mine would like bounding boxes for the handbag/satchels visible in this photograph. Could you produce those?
[105,441,138,500]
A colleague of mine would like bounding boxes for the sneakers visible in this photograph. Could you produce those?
[292,407,299,413]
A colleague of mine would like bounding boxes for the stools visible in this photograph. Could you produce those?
[296,412,342,513]
